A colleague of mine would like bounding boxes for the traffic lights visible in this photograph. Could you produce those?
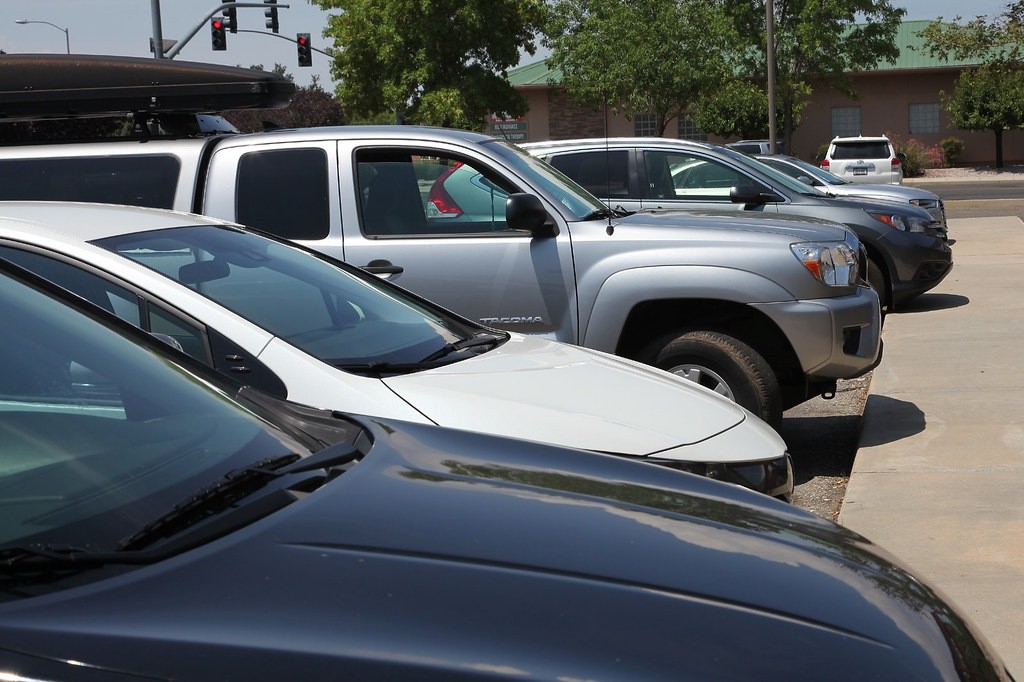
[263,0,280,33]
[296,33,313,66]
[210,17,230,52]
[220,0,237,33]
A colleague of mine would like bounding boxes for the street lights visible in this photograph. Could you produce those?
[14,19,71,54]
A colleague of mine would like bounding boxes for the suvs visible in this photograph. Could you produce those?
[821,132,908,188]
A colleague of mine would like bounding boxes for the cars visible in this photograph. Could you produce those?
[1,257,1017,682]
[712,140,800,162]
[668,156,950,234]
[1,196,794,503]
[425,137,950,303]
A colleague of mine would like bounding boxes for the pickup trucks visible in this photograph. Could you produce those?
[1,120,887,437]
